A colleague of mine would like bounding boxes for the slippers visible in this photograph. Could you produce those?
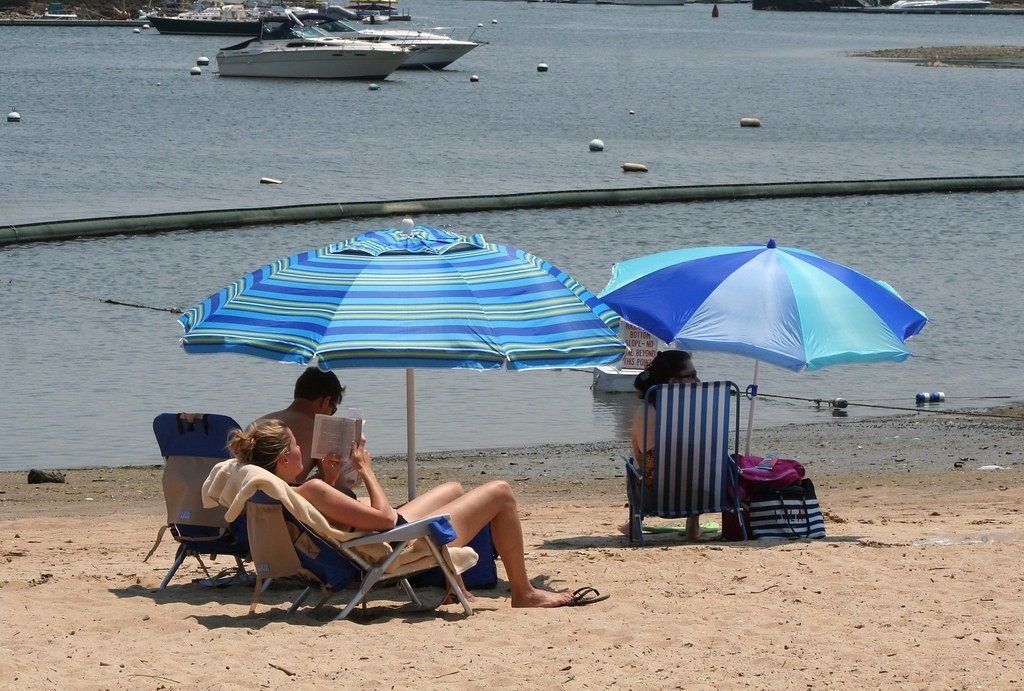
[199,568,258,587]
[558,587,610,606]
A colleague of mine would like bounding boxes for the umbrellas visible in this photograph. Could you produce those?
[175,219,631,500]
[596,238,929,456]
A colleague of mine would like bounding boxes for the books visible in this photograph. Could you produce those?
[310,414,366,463]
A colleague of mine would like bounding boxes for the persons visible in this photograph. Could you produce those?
[245,366,346,481]
[226,419,574,608]
[616,350,704,539]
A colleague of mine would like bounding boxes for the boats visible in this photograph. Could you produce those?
[215,10,416,82]
[139,0,404,37]
[359,26,482,70]
[890,0,991,10]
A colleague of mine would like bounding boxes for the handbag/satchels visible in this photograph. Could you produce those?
[749,478,826,542]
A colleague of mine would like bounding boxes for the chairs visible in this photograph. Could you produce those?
[201,459,480,623]
[622,381,750,544]
[151,412,252,591]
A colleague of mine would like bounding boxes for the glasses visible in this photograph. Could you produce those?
[319,392,337,416]
[667,371,697,382]
